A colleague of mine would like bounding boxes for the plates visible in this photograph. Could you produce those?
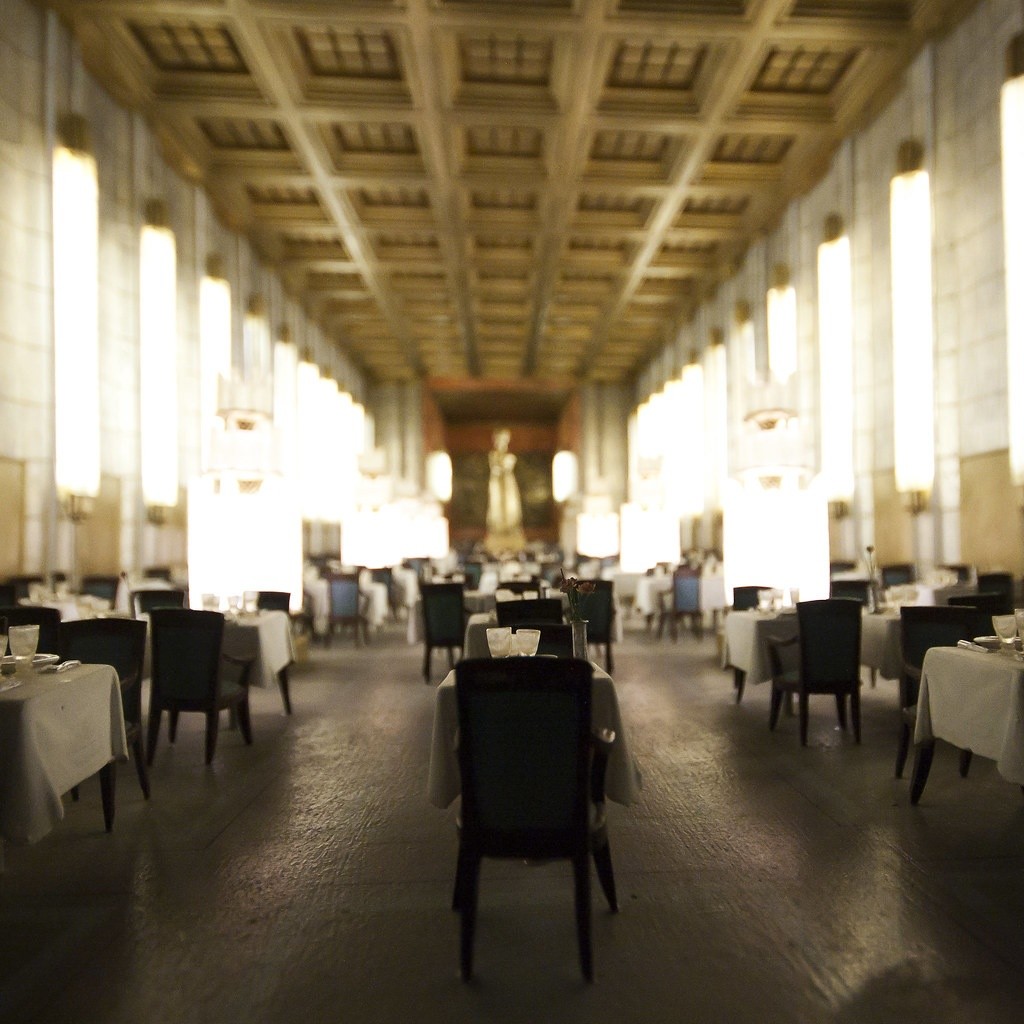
[973,635,1022,650]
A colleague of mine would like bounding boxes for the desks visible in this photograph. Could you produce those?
[718,608,800,730]
[406,588,569,660]
[635,575,725,635]
[314,579,389,641]
[854,611,903,686]
[420,662,642,814]
[142,609,297,746]
[464,613,497,659]
[392,567,419,606]
[916,585,977,609]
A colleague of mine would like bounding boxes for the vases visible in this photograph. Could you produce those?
[568,620,590,661]
[864,581,888,617]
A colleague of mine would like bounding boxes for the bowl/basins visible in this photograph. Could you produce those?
[0,653,60,673]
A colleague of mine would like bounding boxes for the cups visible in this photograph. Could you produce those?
[201,593,220,613]
[486,627,541,657]
[0,624,40,687]
[228,596,238,608]
[757,588,799,613]
[992,608,1024,662]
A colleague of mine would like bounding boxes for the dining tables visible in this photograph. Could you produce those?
[909,646,1024,808]
[0,657,134,863]
[0,550,1024,995]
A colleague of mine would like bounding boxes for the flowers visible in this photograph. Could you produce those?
[556,564,598,619]
[857,543,885,580]
